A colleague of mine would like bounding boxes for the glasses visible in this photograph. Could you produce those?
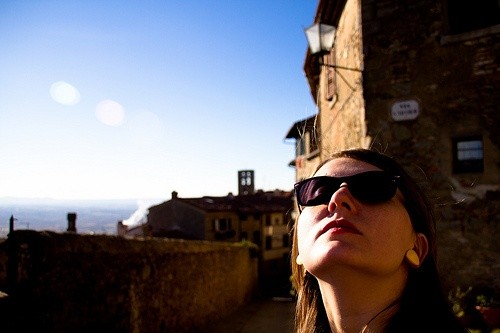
[293,170,424,233]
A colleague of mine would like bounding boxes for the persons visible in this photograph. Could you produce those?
[287,149,492,332]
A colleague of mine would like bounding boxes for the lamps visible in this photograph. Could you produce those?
[306,11,362,92]
